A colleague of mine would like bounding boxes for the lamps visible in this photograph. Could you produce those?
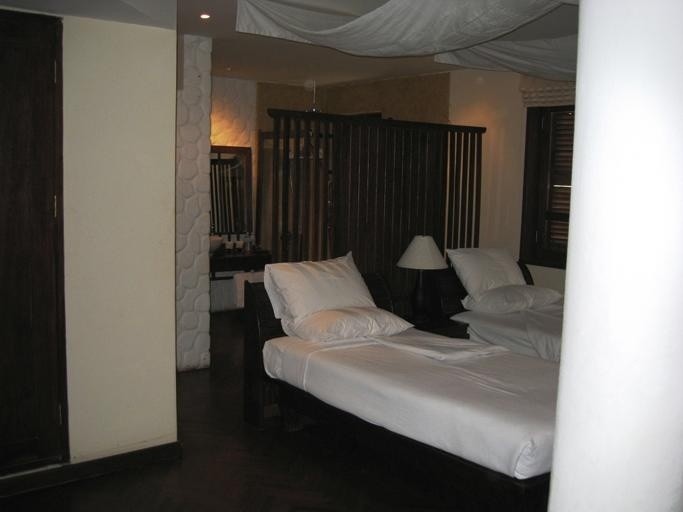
[397,232,449,324]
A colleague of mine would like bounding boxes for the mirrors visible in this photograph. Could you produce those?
[209,145,253,236]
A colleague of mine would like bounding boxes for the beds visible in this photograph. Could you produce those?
[242,263,564,512]
[242,264,563,510]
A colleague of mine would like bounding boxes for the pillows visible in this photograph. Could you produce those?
[446,246,527,298]
[280,308,415,344]
[461,285,562,314]
[265,250,379,317]
